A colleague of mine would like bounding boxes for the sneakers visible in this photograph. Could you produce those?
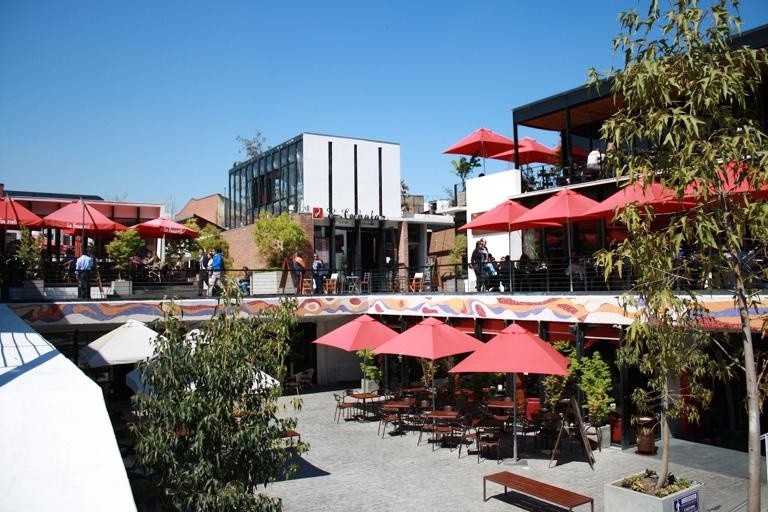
[198,289,216,298]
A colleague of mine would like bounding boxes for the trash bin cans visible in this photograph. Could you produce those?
[525,397,541,422]
[557,399,569,414]
[635,416,656,455]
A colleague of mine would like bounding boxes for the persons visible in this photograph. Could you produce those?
[36,245,159,300]
[311,253,325,295]
[294,252,306,296]
[199,247,253,295]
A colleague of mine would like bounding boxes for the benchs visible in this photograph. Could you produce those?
[482,471,596,512]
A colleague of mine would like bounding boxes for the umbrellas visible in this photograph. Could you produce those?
[448,324,572,465]
[311,313,398,424]
[370,317,485,424]
[130,217,201,259]
[80,319,172,368]
[43,196,137,249]
[0,191,42,244]
[126,329,280,405]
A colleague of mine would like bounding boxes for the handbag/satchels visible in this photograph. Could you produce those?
[318,268,329,277]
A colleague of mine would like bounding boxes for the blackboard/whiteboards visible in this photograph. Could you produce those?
[572,395,596,460]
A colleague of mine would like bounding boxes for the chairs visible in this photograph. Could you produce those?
[299,269,424,293]
[109,406,303,484]
[478,260,628,293]
[330,380,656,465]
[53,258,186,284]
[517,152,602,194]
[284,368,314,396]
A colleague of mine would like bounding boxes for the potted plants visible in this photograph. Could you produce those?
[439,233,469,293]
[601,272,718,511]
[577,350,614,449]
[103,229,147,297]
[11,224,46,300]
[447,148,483,208]
[249,209,307,294]
